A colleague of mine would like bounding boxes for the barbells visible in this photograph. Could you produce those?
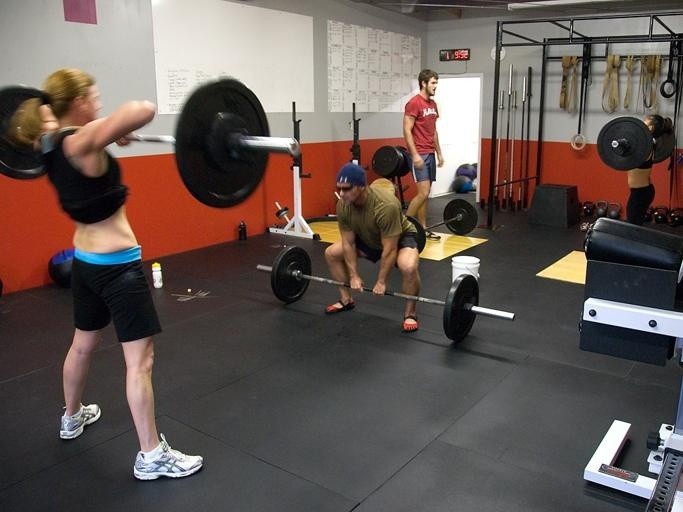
[597,117,675,171]
[0,77,301,208]
[256,245,515,342]
[405,199,477,254]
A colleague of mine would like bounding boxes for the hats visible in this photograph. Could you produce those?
[335,162,366,183]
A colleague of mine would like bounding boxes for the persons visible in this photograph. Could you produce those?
[29,67,203,483]
[624,115,673,228]
[402,68,445,242]
[320,162,423,334]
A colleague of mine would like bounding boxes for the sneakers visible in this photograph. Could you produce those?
[60,403,102,441]
[326,300,356,314]
[133,432,205,480]
[423,229,441,241]
[403,315,418,332]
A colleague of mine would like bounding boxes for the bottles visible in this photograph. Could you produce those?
[152,262,163,289]
[238,221,247,241]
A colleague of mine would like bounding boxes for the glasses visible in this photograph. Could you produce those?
[335,184,354,192]
